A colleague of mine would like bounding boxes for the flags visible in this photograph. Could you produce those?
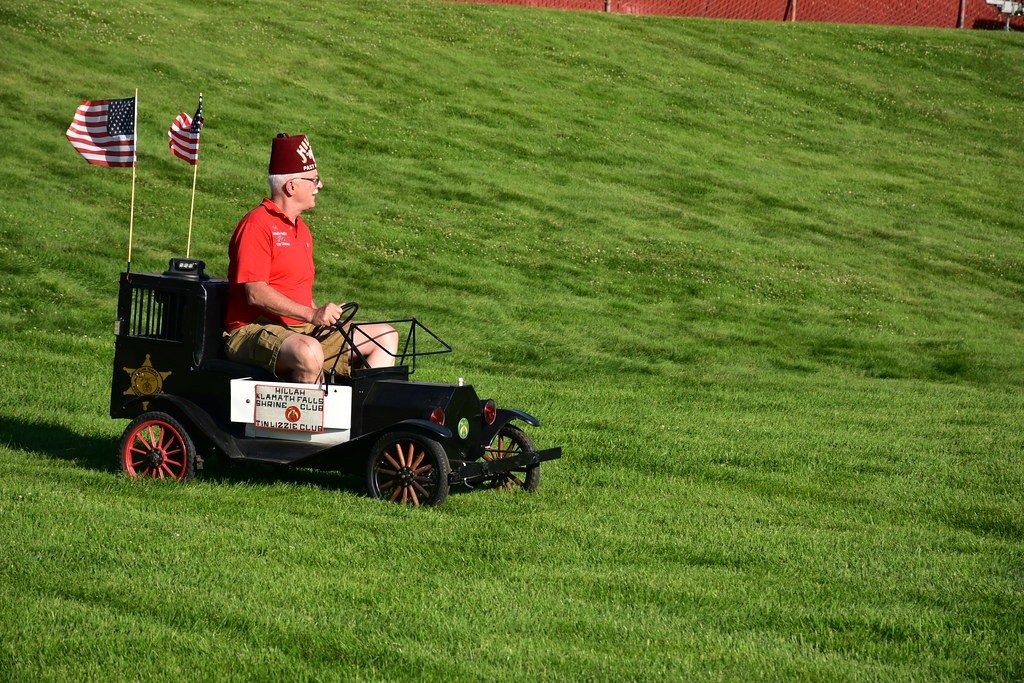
[167,96,205,165]
[66,97,137,167]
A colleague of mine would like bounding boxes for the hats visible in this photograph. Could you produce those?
[269,134,316,175]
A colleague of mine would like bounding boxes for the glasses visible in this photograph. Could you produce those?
[291,176,319,184]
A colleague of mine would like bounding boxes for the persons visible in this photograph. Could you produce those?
[223,156,399,386]
